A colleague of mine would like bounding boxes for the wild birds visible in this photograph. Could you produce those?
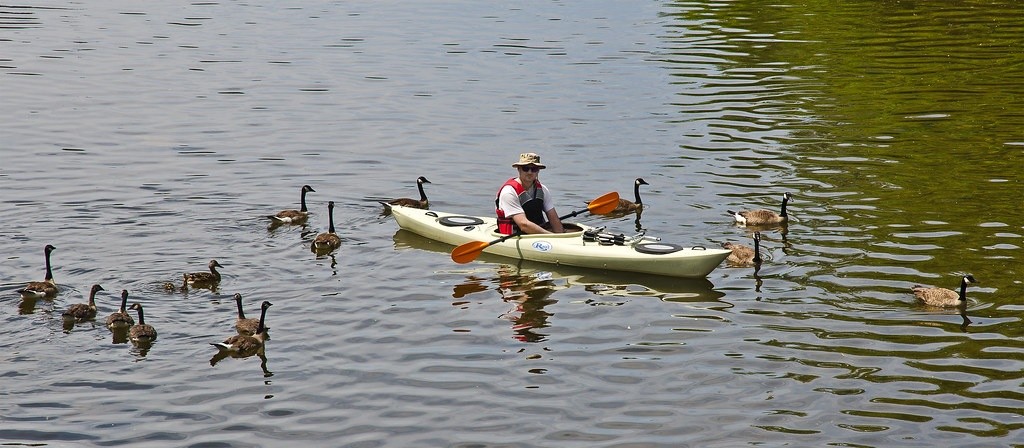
[378,176,431,210]
[910,275,981,307]
[209,300,273,352]
[583,178,650,212]
[13,245,57,299]
[183,260,224,283]
[62,284,105,319]
[106,289,135,329]
[726,192,794,225]
[267,185,316,223]
[310,201,341,250]
[233,293,270,335]
[127,303,157,343]
[721,230,763,264]
[164,275,192,288]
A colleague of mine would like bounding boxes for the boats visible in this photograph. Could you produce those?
[390,204,734,279]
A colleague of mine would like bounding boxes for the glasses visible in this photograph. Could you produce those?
[520,166,539,172]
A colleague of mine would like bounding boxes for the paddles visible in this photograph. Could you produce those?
[451,191,619,264]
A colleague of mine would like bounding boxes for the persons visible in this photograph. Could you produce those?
[494,152,568,235]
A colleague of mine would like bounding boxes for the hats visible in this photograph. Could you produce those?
[511,153,546,169]
